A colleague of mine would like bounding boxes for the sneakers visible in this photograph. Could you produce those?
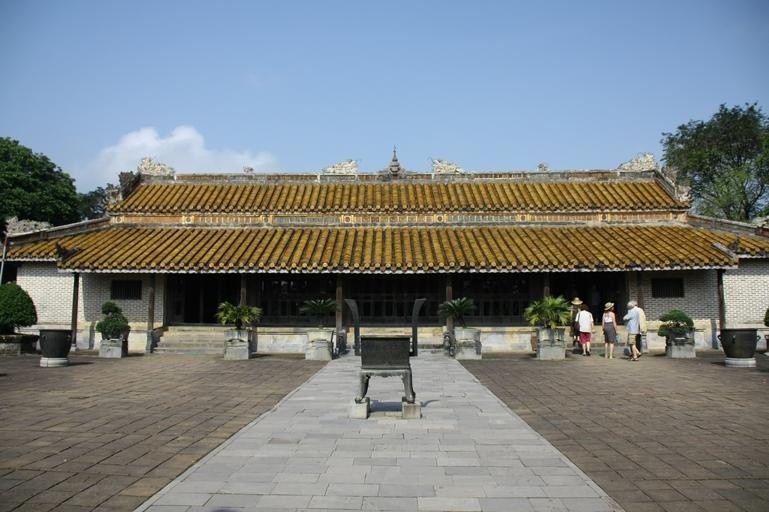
[630,352,642,361]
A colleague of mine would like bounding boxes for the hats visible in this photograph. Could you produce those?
[604,301,615,311]
[571,297,584,305]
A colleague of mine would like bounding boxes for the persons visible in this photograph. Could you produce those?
[570,297,647,361]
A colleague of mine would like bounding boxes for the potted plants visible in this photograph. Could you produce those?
[0,280,38,360]
[652,306,698,363]
[521,293,576,362]
[215,299,264,361]
[95,299,132,360]
[433,295,485,362]
[298,296,342,362]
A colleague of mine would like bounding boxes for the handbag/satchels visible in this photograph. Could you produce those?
[574,322,580,330]
